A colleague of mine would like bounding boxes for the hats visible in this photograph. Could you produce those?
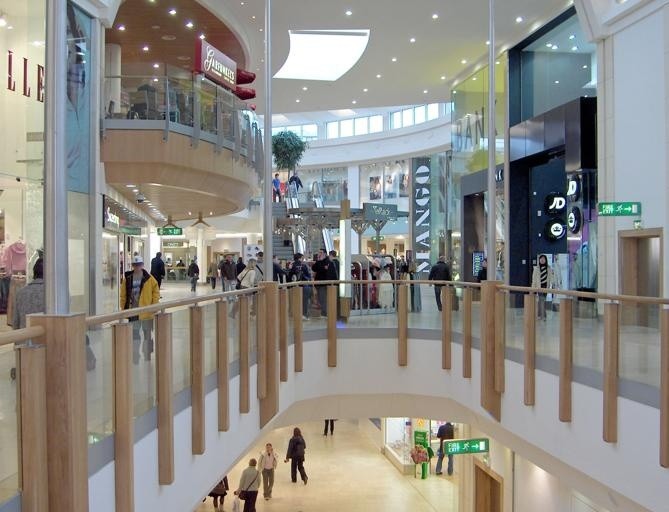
[132,256,143,264]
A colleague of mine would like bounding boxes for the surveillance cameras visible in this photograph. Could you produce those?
[135,193,145,205]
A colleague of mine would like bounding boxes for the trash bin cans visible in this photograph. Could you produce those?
[577,287,597,318]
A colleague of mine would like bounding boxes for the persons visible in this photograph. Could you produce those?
[67,4,89,194]
[119,248,416,364]
[324,420,334,436]
[133,79,156,112]
[477,258,487,290]
[530,255,556,322]
[288,173,303,191]
[236,458,261,512]
[208,476,229,512]
[435,423,454,474]
[257,444,278,500]
[272,173,281,202]
[428,256,453,311]
[283,428,308,485]
[12,257,45,344]
